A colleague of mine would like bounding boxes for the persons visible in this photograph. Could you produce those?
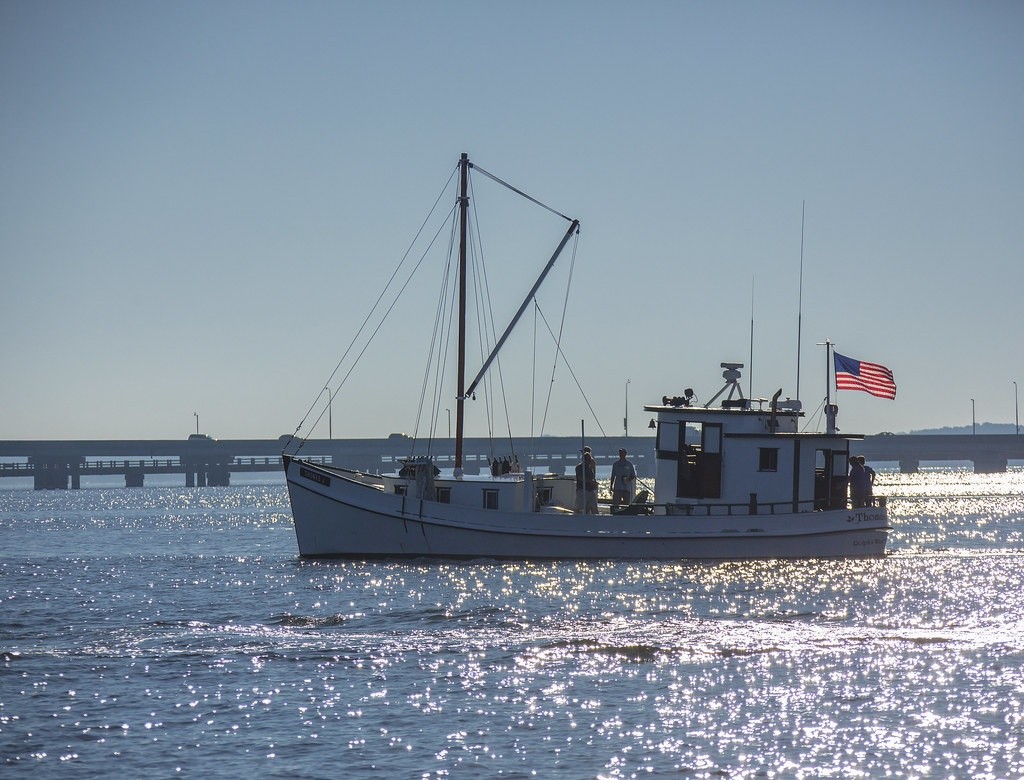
[610,448,635,510]
[575,446,598,514]
[848,455,875,508]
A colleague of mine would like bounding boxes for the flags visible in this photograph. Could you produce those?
[834,351,896,400]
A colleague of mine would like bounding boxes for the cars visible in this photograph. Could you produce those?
[875,432,895,435]
[188,434,218,442]
[686,426,702,434]
[279,434,306,441]
[388,432,415,440]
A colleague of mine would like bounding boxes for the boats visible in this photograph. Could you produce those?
[275,149,895,561]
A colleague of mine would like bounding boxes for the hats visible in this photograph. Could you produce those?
[619,448,627,456]
[579,445,592,452]
[582,452,593,460]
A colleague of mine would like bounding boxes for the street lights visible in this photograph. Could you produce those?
[624,379,631,437]
[1013,381,1019,436]
[970,399,976,435]
[325,386,332,440]
[446,408,451,461]
[193,412,198,435]
